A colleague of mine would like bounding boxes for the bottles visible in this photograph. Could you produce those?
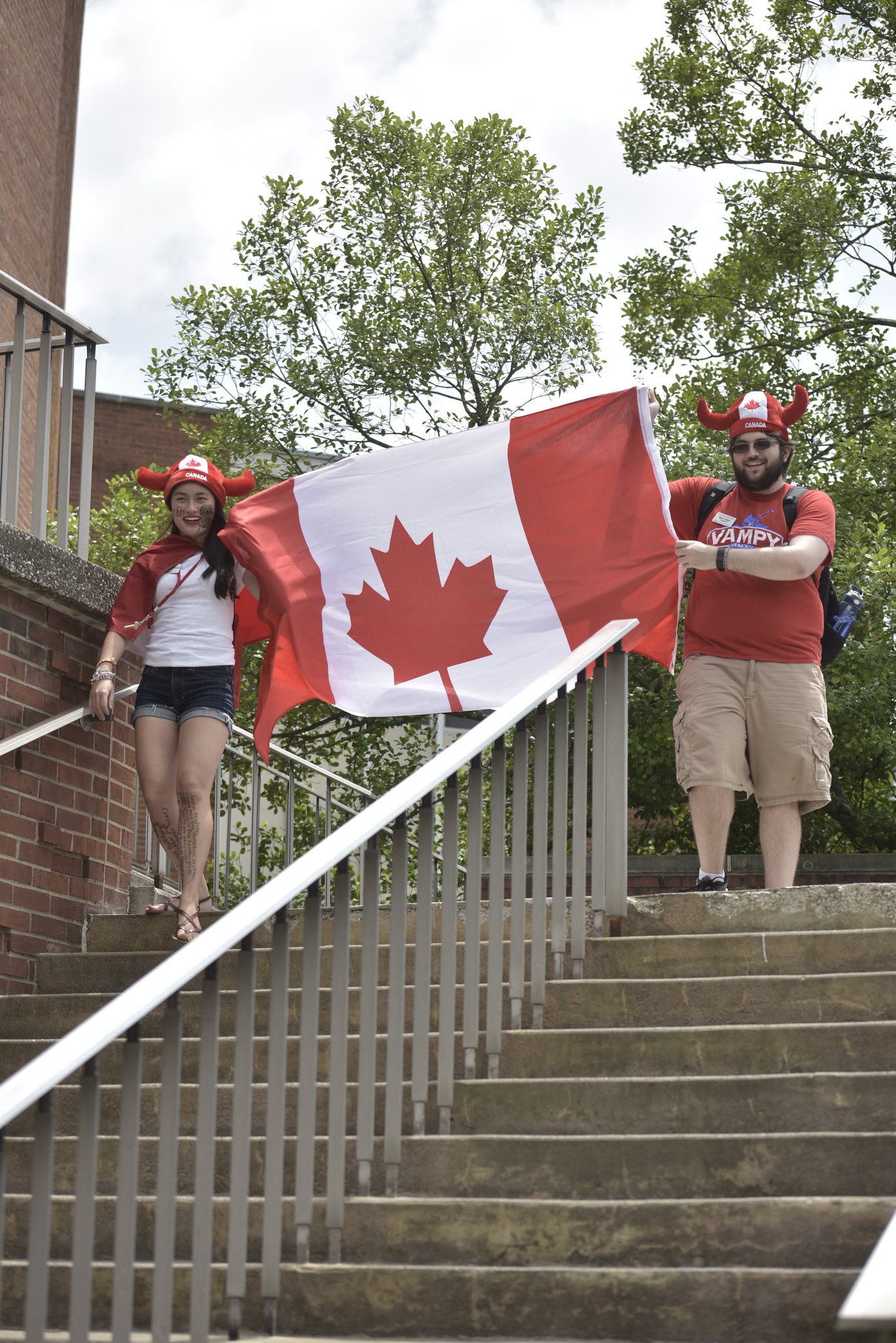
[828,584,865,640]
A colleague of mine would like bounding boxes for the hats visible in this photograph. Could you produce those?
[697,385,809,442]
[136,454,254,509]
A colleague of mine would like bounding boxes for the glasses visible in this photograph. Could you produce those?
[730,440,779,454]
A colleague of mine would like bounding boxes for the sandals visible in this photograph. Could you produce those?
[145,894,212,914]
[171,905,202,944]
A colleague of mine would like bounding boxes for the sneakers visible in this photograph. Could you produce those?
[678,866,728,893]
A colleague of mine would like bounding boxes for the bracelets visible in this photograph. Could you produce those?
[90,669,116,685]
[96,657,117,672]
[716,547,726,572]
[724,548,731,572]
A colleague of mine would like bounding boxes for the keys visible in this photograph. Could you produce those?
[124,621,140,630]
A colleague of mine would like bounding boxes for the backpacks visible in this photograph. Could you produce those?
[694,481,847,665]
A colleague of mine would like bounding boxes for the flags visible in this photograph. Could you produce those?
[215,385,683,764]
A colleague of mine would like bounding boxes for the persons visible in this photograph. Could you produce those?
[641,385,836,892]
[88,454,261,944]
